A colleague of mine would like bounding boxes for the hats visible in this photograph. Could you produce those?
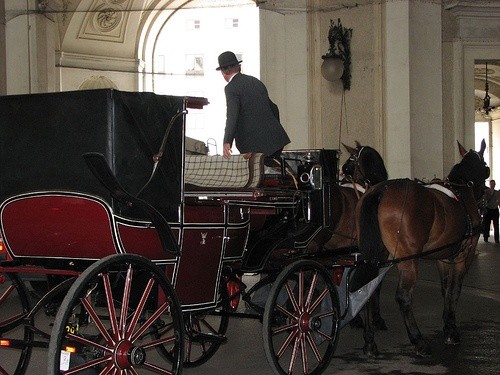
[216,51,242,71]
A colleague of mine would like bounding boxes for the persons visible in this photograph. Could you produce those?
[214,50,291,170]
[482,180,500,244]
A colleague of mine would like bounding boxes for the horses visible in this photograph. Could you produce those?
[355,138,491,360]
[287,141,389,331]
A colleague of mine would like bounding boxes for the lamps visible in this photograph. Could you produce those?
[474,61,500,120]
[320,18,353,91]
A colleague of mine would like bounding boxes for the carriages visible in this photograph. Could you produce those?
[4,88,489,375]
